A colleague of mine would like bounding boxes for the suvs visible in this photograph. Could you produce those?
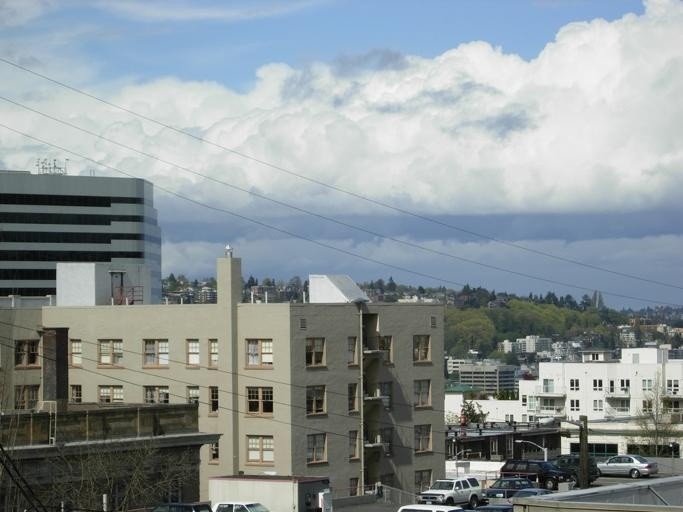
[417,476,484,508]
[499,458,571,490]
[548,452,598,488]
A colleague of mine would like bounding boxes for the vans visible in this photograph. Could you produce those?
[396,502,463,512]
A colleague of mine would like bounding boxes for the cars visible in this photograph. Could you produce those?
[483,478,535,500]
[476,504,513,512]
[597,454,659,478]
[150,501,213,512]
[212,501,271,512]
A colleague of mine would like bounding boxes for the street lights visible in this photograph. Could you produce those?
[513,439,549,461]
[537,416,584,491]
[455,448,472,478]
